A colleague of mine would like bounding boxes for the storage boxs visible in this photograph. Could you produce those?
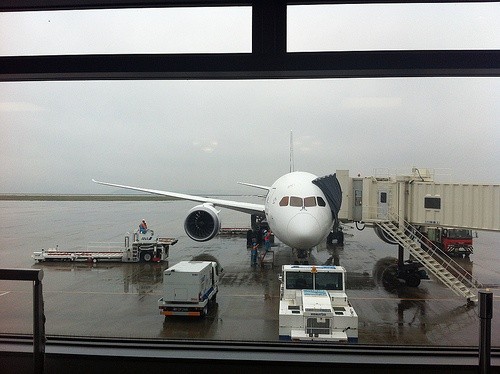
[162,259,215,303]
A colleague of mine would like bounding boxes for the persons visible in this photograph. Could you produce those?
[263,230,271,252]
[251,243,260,267]
[139,219,148,235]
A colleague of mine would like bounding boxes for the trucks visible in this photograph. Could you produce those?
[411,225,478,257]
[158,261,224,320]
[278,265,358,342]
[31,229,178,262]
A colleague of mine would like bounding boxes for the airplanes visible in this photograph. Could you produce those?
[90,132,343,269]
